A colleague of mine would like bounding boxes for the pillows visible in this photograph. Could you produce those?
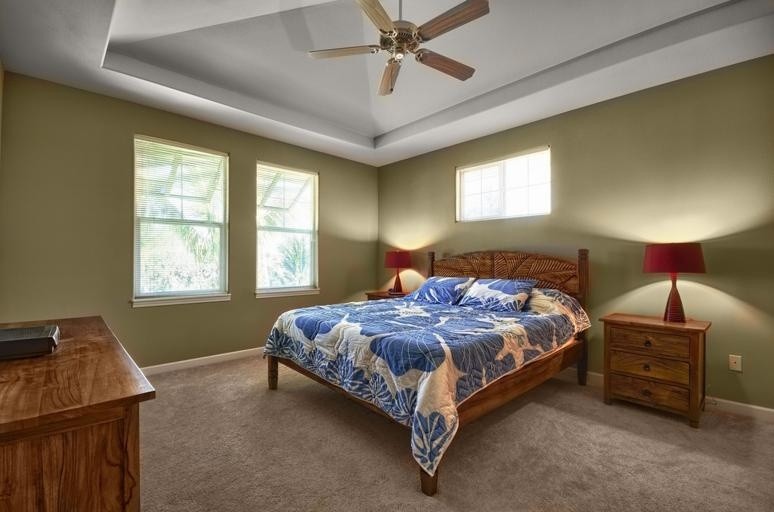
[407,274,540,314]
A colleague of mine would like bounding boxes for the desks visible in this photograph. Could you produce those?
[1,315,156,512]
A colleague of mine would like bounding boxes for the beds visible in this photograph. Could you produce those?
[261,249,593,496]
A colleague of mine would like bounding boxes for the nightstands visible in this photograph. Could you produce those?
[599,312,711,427]
[365,291,411,301]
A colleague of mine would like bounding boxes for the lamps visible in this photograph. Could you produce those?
[394,44,406,61]
[642,241,707,322]
[383,251,413,294]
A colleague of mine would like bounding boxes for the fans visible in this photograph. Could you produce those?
[307,0,491,97]
